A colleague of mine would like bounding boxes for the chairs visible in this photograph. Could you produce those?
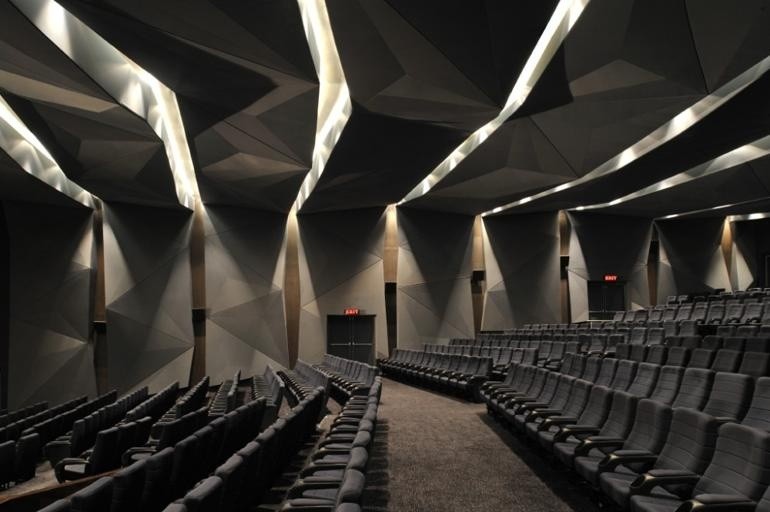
[0,353,383,512]
[375,287,769,405]
[480,325,770,512]
